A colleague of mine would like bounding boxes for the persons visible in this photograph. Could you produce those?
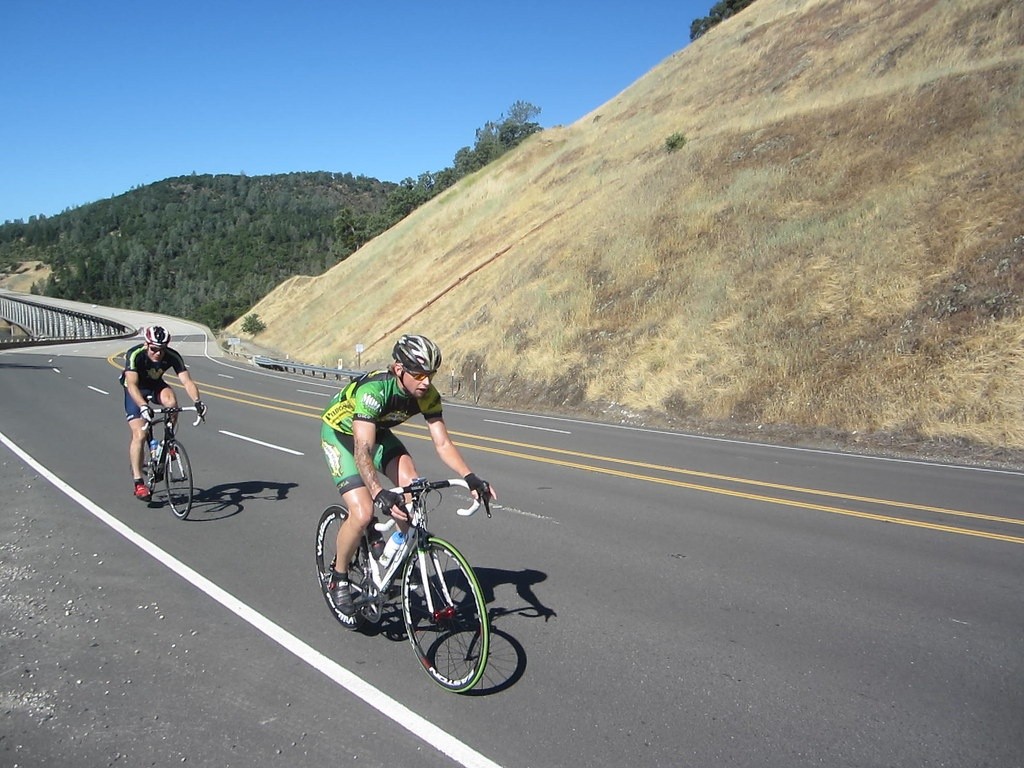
[323,334,493,616]
[122,325,205,501]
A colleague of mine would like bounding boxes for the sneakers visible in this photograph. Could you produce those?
[326,568,360,630]
[133,485,152,502]
[169,449,177,460]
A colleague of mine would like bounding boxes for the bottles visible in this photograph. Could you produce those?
[367,514,387,560]
[150,438,159,461]
[156,439,165,460]
[378,530,405,567]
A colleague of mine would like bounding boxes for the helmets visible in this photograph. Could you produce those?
[145,325,171,348]
[392,335,442,375]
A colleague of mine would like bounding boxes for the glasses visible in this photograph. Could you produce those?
[150,346,168,352]
[402,366,437,381]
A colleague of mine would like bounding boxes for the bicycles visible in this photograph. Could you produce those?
[315,477,492,695]
[129,392,207,520]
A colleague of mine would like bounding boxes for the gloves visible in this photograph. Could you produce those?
[194,399,207,417]
[140,405,154,421]
